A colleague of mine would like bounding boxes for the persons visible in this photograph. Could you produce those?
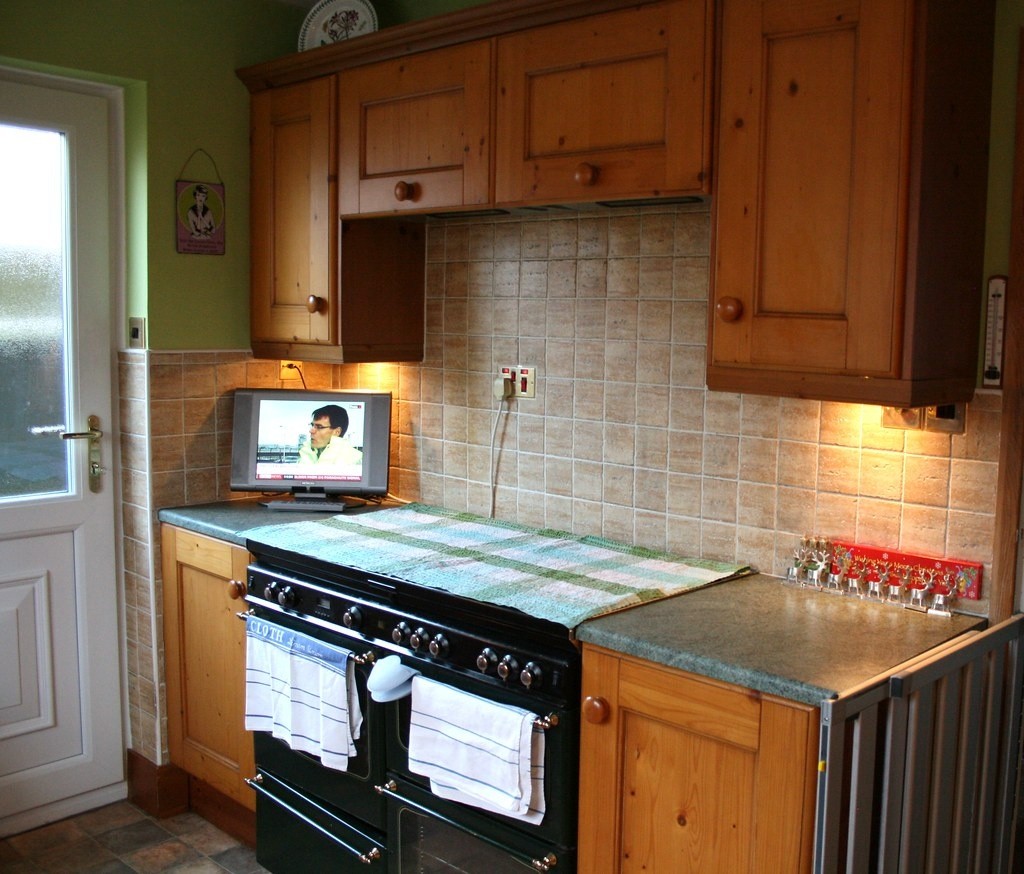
[296,405,362,477]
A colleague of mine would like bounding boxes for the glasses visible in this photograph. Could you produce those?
[308,422,335,431]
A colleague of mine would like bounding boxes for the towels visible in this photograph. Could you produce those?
[245,613,364,772]
[407,675,546,826]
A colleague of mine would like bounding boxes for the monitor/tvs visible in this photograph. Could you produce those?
[230,387,393,507]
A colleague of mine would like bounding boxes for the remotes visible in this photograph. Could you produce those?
[268,500,346,511]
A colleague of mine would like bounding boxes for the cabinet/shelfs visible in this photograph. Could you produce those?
[337,0,717,222]
[707,0,997,407]
[249,73,426,365]
[577,636,887,874]
[159,523,266,850]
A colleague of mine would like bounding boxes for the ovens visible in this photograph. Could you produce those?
[242,537,583,874]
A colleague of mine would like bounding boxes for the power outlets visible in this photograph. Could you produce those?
[499,365,536,400]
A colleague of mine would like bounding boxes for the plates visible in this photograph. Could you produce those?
[297,0,380,56]
